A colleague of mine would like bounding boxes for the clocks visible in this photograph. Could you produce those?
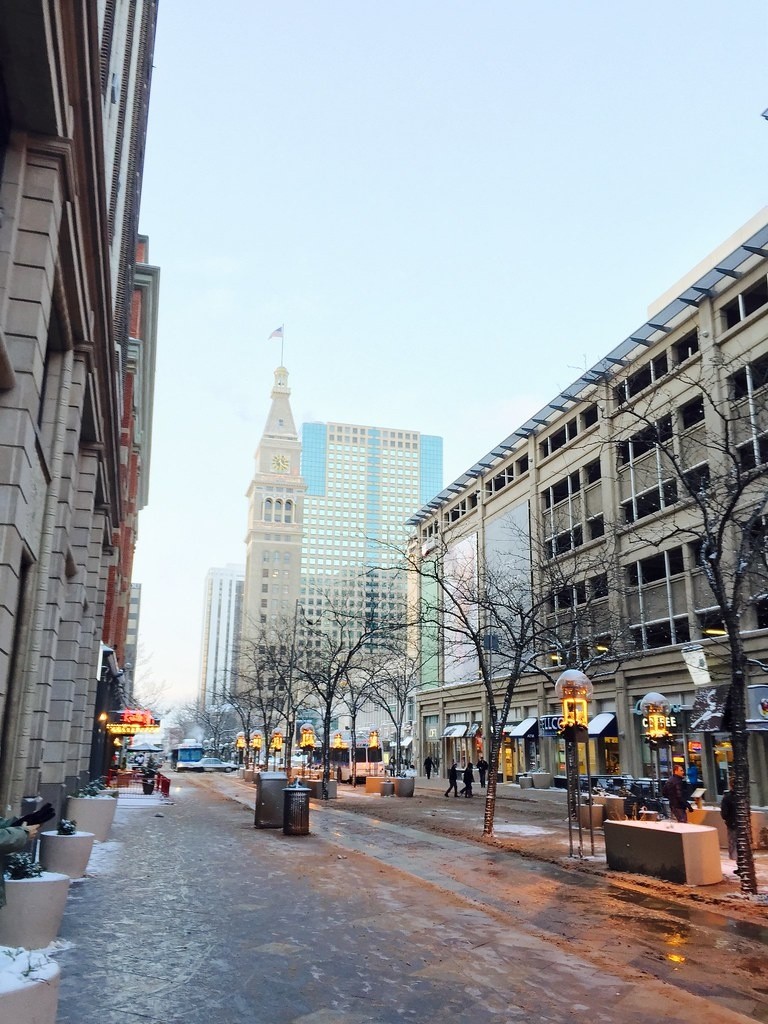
[271,452,291,474]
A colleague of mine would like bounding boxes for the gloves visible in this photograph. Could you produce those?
[10,803,56,827]
[688,806,693,812]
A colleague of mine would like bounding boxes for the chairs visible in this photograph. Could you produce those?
[117,773,130,787]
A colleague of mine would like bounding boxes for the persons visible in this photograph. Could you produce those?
[444,757,489,798]
[0,811,45,910]
[662,761,700,823]
[423,754,436,780]
[388,753,408,777]
[128,752,145,766]
[720,775,738,860]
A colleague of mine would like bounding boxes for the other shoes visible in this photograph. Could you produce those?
[445,794,449,797]
[455,796,460,797]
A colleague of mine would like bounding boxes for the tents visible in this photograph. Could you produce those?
[126,742,164,753]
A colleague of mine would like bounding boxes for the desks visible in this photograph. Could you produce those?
[122,770,142,782]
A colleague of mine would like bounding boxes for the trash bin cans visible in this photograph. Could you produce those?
[254,771,289,830]
[281,787,312,836]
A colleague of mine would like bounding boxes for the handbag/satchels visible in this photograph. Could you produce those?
[388,764,393,769]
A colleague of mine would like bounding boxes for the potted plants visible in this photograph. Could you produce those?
[141,756,162,794]
[0,944,60,1024]
[576,792,658,829]
[519,768,551,789]
[66,776,118,842]
[40,819,96,878]
[0,850,72,952]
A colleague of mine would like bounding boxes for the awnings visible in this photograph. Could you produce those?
[509,718,538,738]
[587,713,616,736]
[400,736,414,746]
[440,722,482,738]
